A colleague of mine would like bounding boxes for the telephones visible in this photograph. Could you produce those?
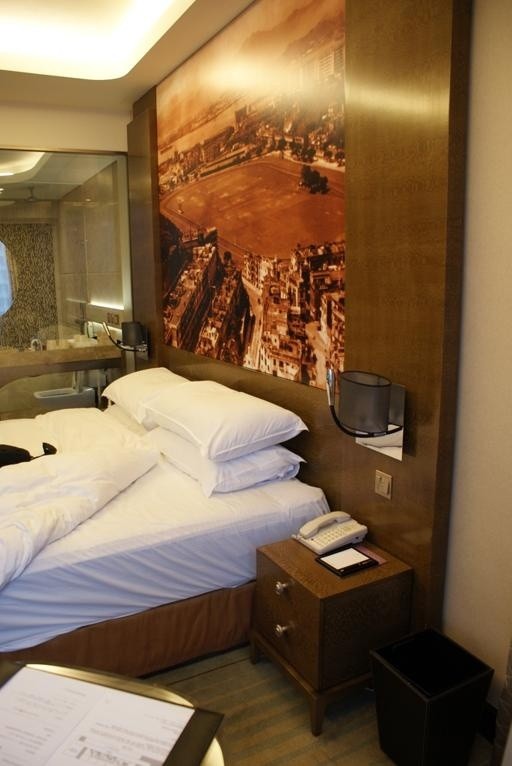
[296,511,368,555]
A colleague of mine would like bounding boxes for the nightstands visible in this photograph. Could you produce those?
[249,539,415,737]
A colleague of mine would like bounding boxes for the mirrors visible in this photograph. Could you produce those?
[0,147,129,355]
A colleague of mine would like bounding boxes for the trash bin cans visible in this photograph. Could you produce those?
[368,627,495,766]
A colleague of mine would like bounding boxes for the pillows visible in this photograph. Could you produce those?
[102,367,310,498]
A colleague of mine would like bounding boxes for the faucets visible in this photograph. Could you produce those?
[31,338,43,352]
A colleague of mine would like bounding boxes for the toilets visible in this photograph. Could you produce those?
[33,369,106,410]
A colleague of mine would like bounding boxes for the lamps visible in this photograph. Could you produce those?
[103,320,149,353]
[325,369,406,439]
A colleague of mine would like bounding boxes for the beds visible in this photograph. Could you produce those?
[0,406,332,679]
